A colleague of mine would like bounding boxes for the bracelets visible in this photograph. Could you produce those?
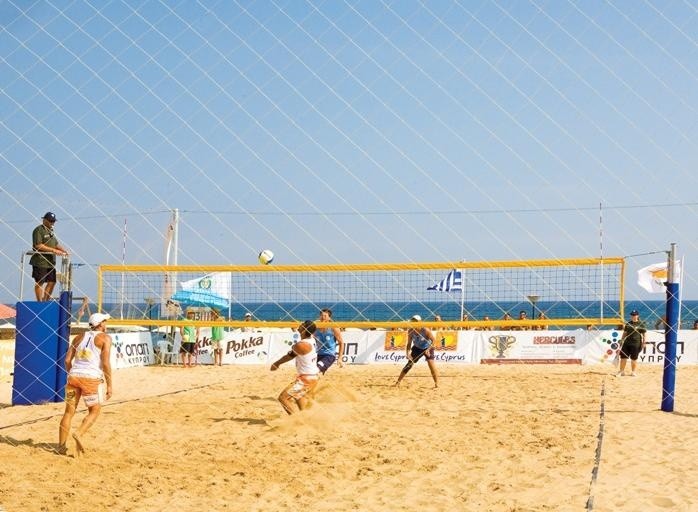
[272,363,277,368]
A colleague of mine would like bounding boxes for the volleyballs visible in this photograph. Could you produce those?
[258,250,274,263]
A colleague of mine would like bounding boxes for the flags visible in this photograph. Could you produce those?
[158,206,178,317]
[171,269,229,307]
[426,262,463,292]
[637,258,681,295]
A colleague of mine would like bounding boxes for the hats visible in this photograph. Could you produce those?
[89,313,110,327]
[410,315,421,321]
[629,310,639,315]
[41,211,58,221]
[245,313,251,317]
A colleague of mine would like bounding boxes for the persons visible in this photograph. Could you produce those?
[270,320,320,417]
[56,312,113,455]
[312,307,347,370]
[179,308,195,367]
[654,313,666,330]
[614,309,647,378]
[691,320,698,329]
[210,310,225,366]
[239,313,256,333]
[429,313,550,331]
[29,212,68,303]
[394,315,440,389]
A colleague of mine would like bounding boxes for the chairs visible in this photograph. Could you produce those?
[155,341,179,367]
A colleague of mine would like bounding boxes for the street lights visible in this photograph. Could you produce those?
[526,292,543,321]
[144,296,155,331]
